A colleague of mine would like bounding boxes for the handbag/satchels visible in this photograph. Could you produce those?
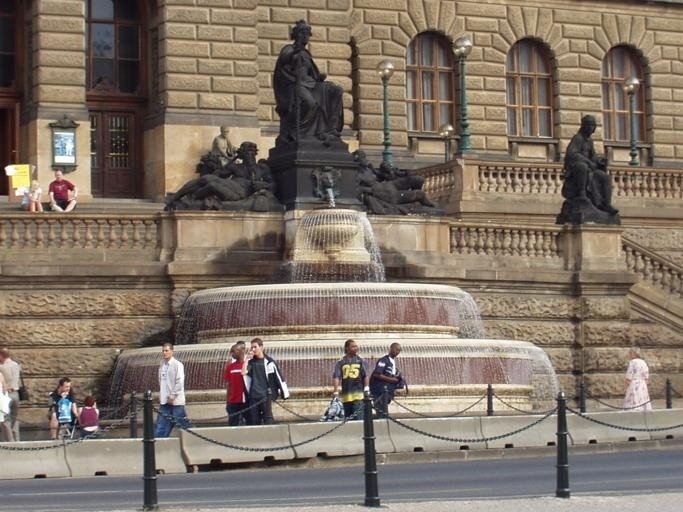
[17,386,30,402]
[319,395,346,422]
[368,368,389,394]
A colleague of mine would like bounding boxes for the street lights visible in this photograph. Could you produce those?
[438,123,454,161]
[453,36,473,153]
[621,75,640,165]
[375,58,396,180]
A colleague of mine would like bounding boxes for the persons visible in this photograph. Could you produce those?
[353,149,439,209]
[623,345,652,411]
[273,17,348,142]
[47,169,79,212]
[211,125,238,166]
[223,344,252,425]
[241,337,290,424]
[154,341,195,437]
[164,140,278,212]
[48,376,79,438]
[369,158,416,181]
[21,179,44,212]
[232,340,257,425]
[370,342,402,418]
[0,374,12,424]
[332,337,370,420]
[77,396,101,439]
[559,114,621,216]
[0,346,23,441]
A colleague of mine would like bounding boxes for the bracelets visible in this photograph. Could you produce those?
[333,392,339,394]
[363,386,370,391]
